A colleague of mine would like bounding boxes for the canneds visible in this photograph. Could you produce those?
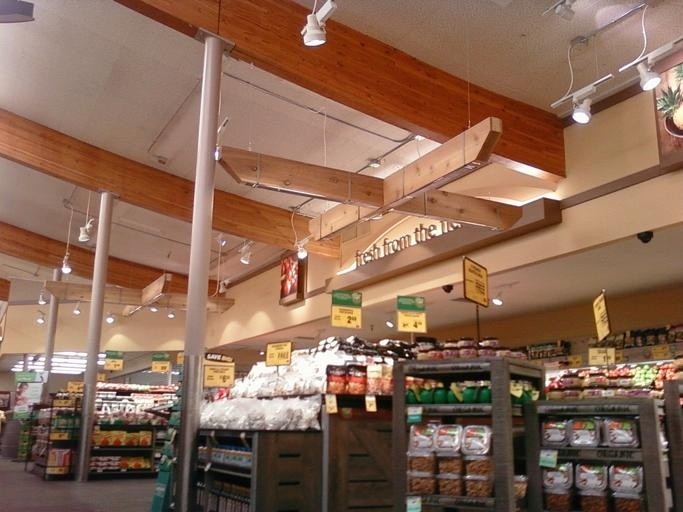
[416,336,510,359]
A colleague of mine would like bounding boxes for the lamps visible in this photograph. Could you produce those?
[104,301,176,326]
[548,36,598,125]
[214,114,310,294]
[619,4,662,94]
[555,0,576,22]
[301,1,328,48]
[34,186,96,325]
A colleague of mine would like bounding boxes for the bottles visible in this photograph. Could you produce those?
[196,479,249,512]
[196,446,252,470]
